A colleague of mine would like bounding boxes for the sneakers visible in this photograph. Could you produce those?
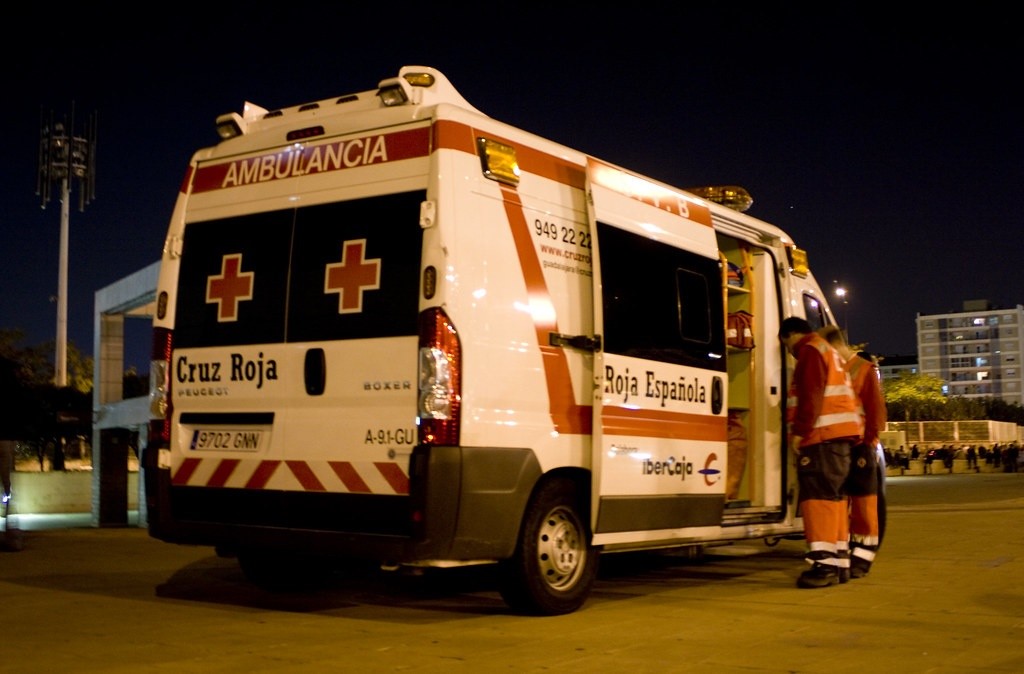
[797,564,839,586]
[838,568,851,582]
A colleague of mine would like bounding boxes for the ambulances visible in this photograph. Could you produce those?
[138,64,845,618]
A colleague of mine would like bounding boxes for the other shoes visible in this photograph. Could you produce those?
[851,567,865,577]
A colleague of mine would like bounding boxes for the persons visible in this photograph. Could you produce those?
[37,434,86,471]
[778,317,865,590]
[883,442,1024,476]
[818,326,887,578]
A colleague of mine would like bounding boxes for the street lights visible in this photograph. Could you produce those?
[38,122,99,470]
